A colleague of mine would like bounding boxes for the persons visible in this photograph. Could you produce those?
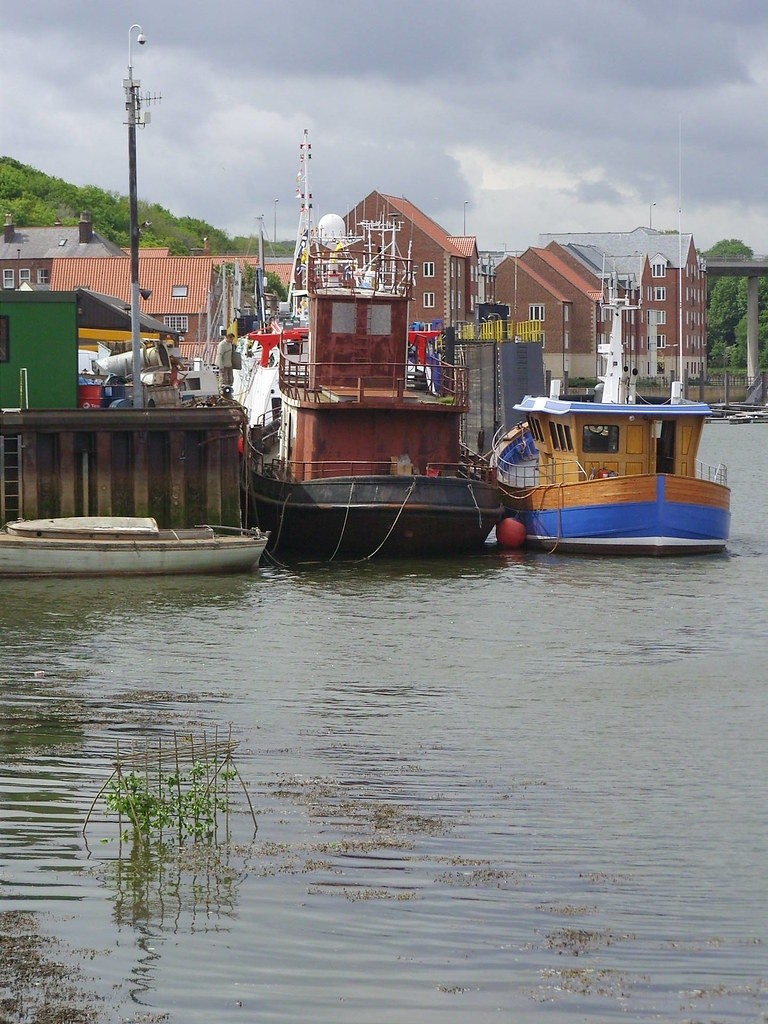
[213,333,237,400]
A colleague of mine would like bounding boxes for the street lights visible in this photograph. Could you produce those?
[649,202,656,229]
[123,23,151,408]
[274,197,280,253]
[464,200,470,236]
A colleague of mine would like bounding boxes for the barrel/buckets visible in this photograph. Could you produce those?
[102,385,126,409]
[79,385,100,409]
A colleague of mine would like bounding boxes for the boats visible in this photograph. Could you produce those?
[232,128,505,553]
[491,254,734,553]
[2,517,271,572]
